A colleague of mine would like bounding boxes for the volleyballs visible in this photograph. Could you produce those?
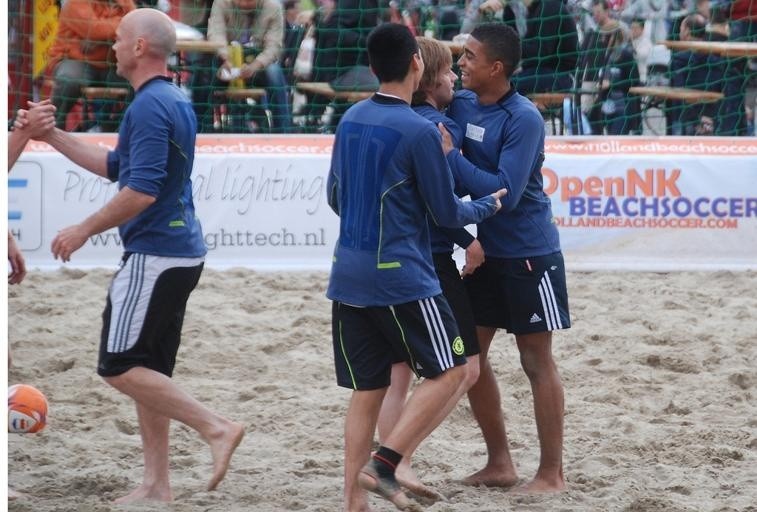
[8,384,48,433]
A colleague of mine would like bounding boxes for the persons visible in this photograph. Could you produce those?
[326,23,509,511]
[9,2,757,135]
[379,35,486,500]
[9,8,246,501]
[9,99,58,286]
[437,21,570,495]
